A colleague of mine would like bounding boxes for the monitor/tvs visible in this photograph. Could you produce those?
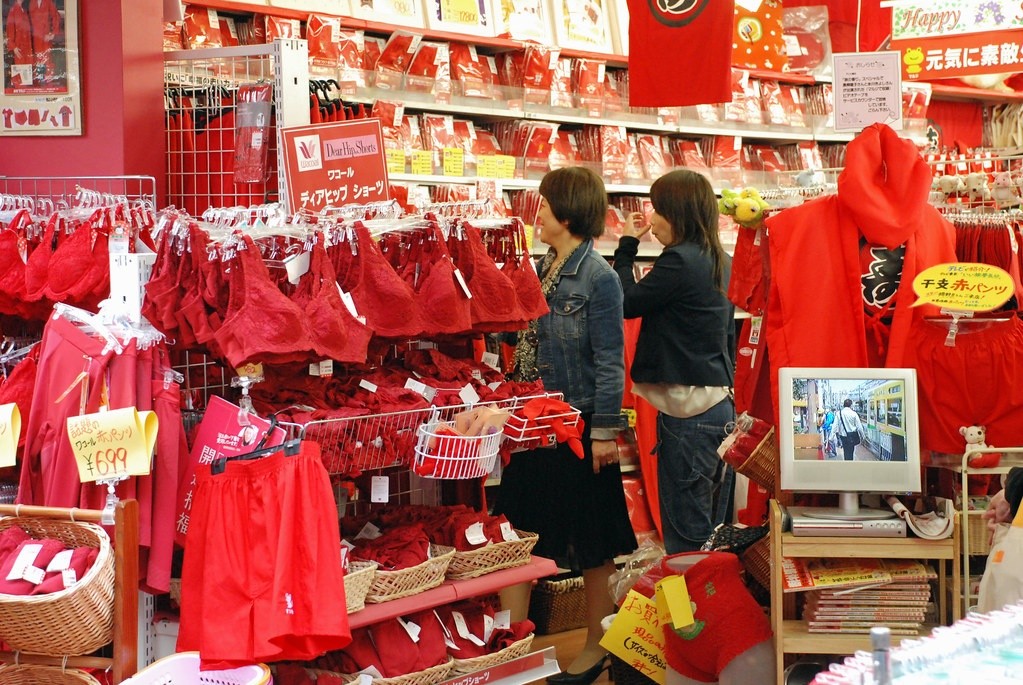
[778,366,922,520]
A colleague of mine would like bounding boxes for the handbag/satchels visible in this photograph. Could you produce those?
[846,430,861,445]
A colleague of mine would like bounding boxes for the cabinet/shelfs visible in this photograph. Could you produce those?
[176,1,931,330]
[768,506,961,685]
[925,445,1023,617]
[155,558,562,684]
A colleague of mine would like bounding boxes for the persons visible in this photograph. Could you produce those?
[611,169,744,557]
[828,398,866,462]
[7,0,61,85]
[978,466,1023,615]
[816,406,838,458]
[497,168,638,685]
[657,548,778,685]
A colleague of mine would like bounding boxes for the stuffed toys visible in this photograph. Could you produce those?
[959,426,1000,469]
[990,170,1023,205]
[963,171,991,200]
[792,168,818,187]
[938,173,965,195]
[718,185,769,227]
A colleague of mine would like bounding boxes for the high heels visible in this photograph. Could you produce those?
[545,653,613,685]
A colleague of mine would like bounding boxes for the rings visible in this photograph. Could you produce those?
[608,460,613,464]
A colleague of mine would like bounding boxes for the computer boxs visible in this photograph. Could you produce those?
[786,506,907,539]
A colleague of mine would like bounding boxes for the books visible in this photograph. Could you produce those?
[887,495,955,541]
[778,558,938,640]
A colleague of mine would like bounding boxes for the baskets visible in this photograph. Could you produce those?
[1,662,100,685]
[270,632,534,684]
[341,529,540,616]
[0,516,116,657]
[743,531,771,595]
[529,576,591,633]
[117,648,274,685]
[933,573,978,622]
[734,423,776,495]
[961,515,994,556]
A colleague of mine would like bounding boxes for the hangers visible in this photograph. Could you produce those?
[0,179,512,474]
